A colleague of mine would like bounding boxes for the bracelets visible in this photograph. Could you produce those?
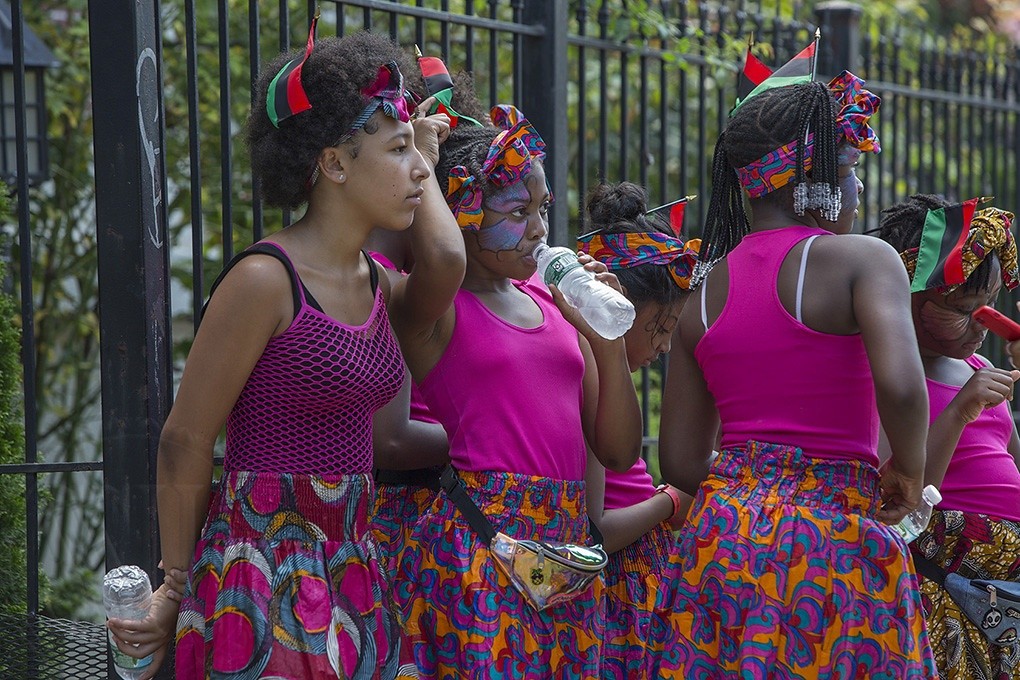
[653,484,680,521]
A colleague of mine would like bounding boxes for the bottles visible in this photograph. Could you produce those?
[887,484,942,545]
[530,243,636,341]
[103,564,153,680]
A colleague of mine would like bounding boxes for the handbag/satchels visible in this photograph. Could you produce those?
[914,553,1020,647]
[437,464,608,608]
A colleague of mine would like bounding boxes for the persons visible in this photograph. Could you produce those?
[106,31,449,680]
[860,194,1020,680]
[576,173,694,680]
[640,83,938,680]
[387,62,641,680]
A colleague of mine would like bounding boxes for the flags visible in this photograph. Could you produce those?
[652,202,685,238]
[420,56,485,128]
[730,41,815,119]
[266,18,314,128]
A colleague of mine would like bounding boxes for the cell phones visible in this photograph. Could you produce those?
[972,305,1020,341]
